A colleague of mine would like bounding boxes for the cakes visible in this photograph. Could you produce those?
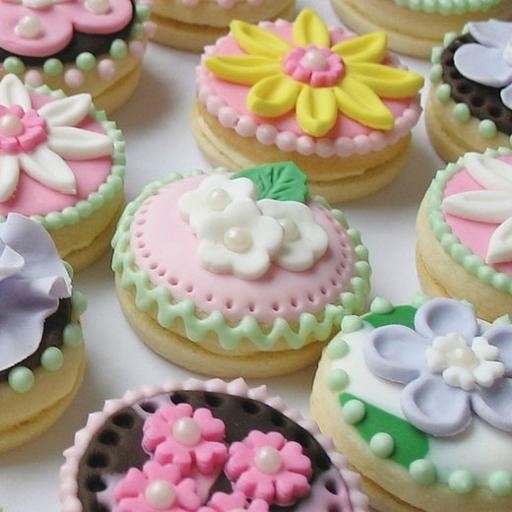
[1,71,126,272]
[329,0,512,58]
[62,375,369,512]
[1,213,88,453]
[308,292,511,511]
[136,0,298,54]
[412,144,511,325]
[421,18,511,161]
[111,163,370,380]
[1,0,158,116]
[191,6,425,202]
